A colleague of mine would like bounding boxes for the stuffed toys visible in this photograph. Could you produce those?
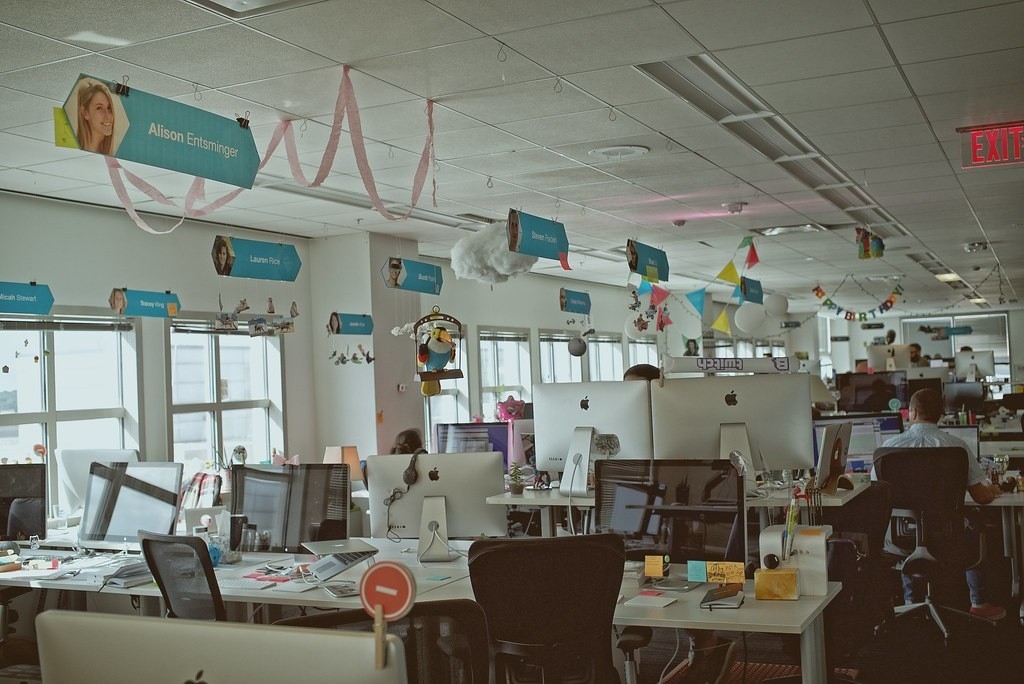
[416,326,457,396]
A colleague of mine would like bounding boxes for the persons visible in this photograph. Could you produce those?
[329,313,340,334]
[910,344,929,367]
[110,291,127,312]
[77,81,118,157]
[624,364,664,380]
[386,259,402,286]
[507,210,519,251]
[871,389,1007,621]
[390,428,422,455]
[214,240,232,274]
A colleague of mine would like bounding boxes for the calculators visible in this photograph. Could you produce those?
[325,585,359,597]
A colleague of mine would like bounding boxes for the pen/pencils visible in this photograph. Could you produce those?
[709,605,712,612]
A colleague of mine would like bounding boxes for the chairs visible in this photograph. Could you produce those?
[176,472,225,535]
[870,445,1001,646]
[437,533,653,683]
[8,497,43,541]
[138,529,228,621]
[270,597,490,684]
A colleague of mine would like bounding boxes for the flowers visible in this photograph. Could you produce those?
[203,443,231,470]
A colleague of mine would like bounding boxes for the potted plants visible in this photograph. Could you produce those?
[508,460,525,494]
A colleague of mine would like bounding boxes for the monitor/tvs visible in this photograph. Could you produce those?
[54,449,142,516]
[77,462,184,555]
[230,345,994,591]
[0,464,46,542]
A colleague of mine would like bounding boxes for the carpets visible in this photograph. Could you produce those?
[659,657,862,684]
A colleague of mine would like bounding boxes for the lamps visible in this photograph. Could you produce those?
[321,445,366,512]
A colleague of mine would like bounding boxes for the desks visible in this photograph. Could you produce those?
[0,420,1024,684]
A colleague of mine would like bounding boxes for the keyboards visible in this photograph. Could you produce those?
[215,579,277,590]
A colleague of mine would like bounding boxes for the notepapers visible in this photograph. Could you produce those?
[425,575,452,580]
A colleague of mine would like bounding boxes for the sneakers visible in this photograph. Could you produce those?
[970,607,1007,620]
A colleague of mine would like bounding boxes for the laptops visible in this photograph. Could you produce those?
[301,539,379,582]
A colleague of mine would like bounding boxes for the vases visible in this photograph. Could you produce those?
[220,468,232,511]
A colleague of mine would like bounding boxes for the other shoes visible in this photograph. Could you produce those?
[677,638,736,684]
